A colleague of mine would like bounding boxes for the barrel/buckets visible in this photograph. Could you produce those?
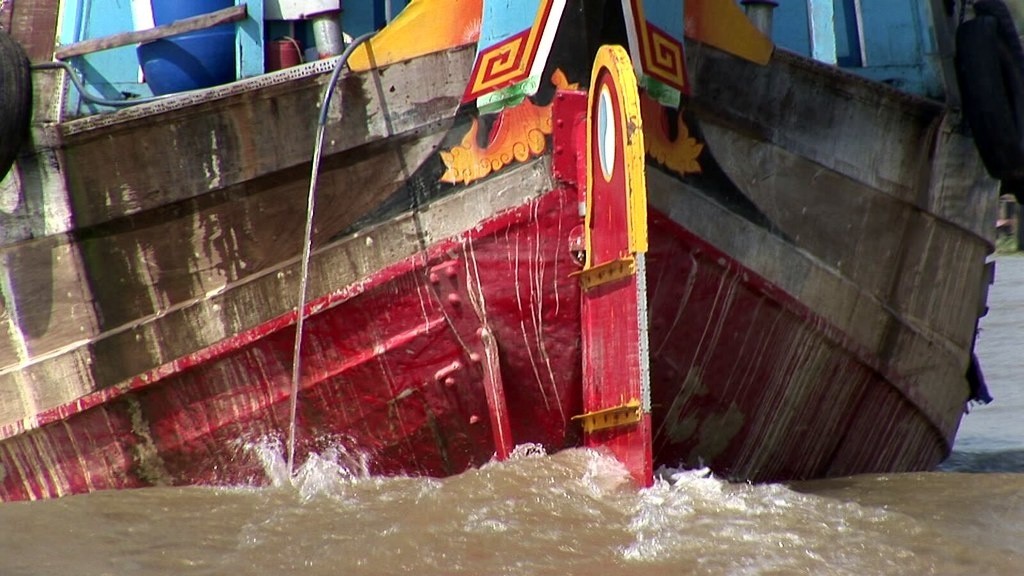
[264,41,299,73]
[130,0,236,96]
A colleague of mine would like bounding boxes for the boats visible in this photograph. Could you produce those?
[0,1,1024,485]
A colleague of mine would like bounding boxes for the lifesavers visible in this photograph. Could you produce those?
[954,14,1023,186]
[1,22,33,180]
